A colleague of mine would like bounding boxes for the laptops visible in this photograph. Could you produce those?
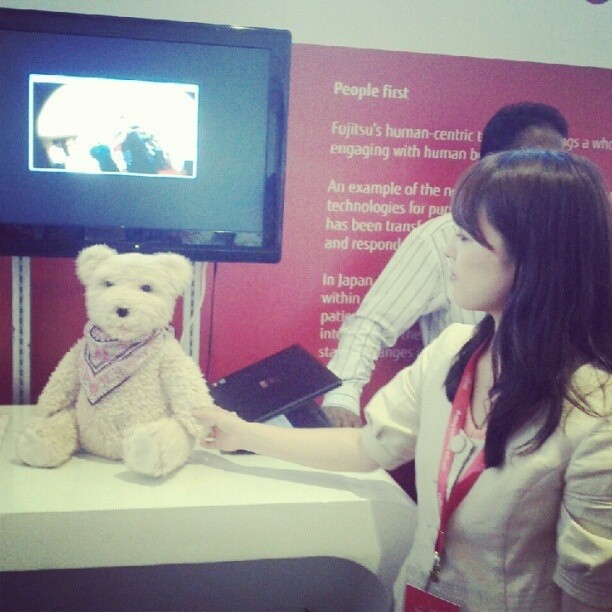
[205,343,342,455]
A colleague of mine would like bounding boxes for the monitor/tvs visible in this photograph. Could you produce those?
[0,6,291,264]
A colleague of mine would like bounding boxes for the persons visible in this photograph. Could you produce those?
[321,103,571,428]
[191,149,612,612]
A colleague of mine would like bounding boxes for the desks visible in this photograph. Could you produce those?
[1,405,419,587]
